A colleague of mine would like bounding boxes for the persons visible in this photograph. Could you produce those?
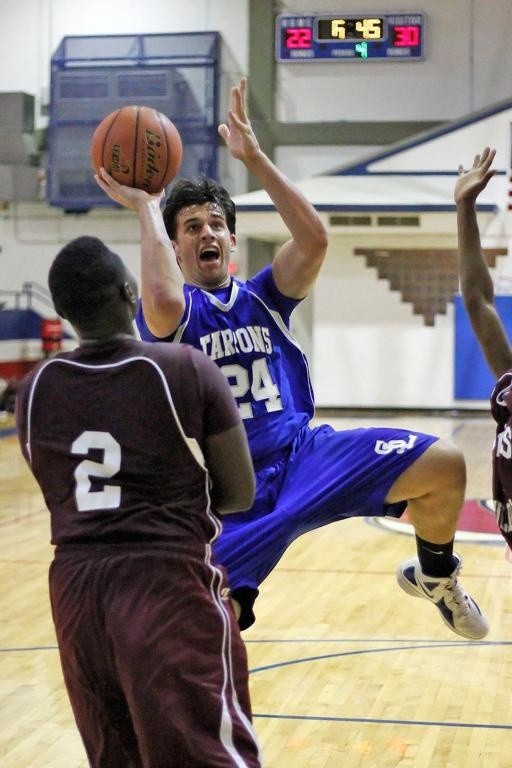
[13,234,270,768]
[451,145,512,556]
[92,72,492,643]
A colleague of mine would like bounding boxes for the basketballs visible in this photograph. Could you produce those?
[91,106,183,194]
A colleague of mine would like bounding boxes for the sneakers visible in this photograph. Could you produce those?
[396,551,490,640]
